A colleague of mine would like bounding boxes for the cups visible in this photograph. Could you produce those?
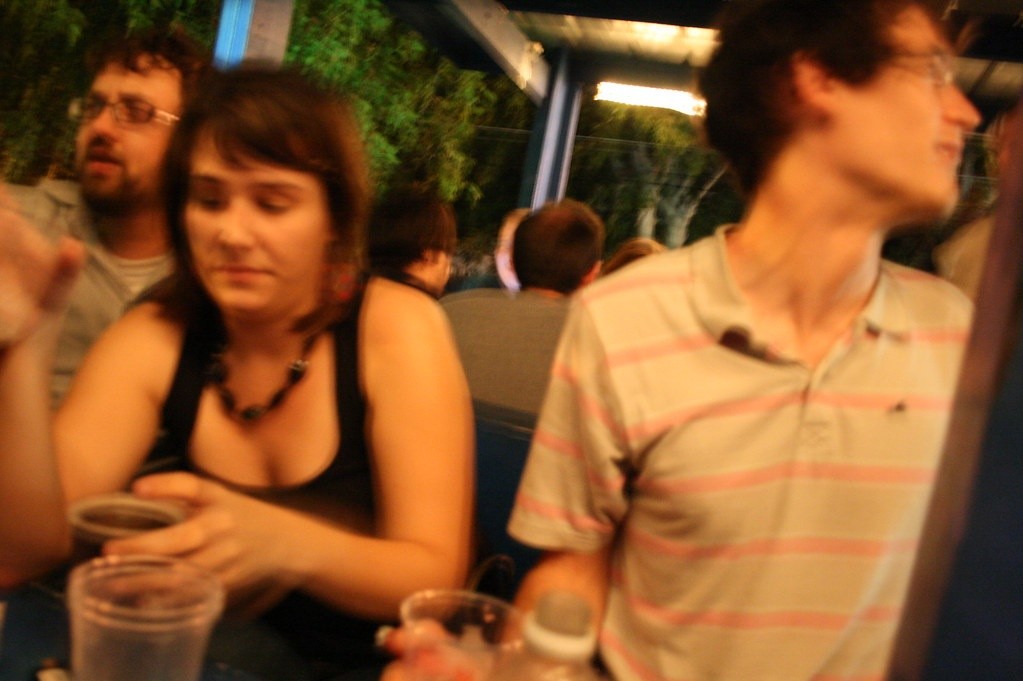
[63,495,191,581]
[65,555,225,681]
[400,586,527,679]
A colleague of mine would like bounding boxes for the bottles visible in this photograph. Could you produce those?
[504,594,601,680]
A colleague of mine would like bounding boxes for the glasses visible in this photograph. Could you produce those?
[67,93,180,126]
[876,50,955,88]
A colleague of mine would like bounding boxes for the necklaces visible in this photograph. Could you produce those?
[214,330,319,427]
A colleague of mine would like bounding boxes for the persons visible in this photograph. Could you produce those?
[435,197,671,412]
[371,0,997,681]
[928,110,1022,680]
[1,27,217,425]
[682,0,983,279]
[366,184,456,301]
[1,54,478,681]
